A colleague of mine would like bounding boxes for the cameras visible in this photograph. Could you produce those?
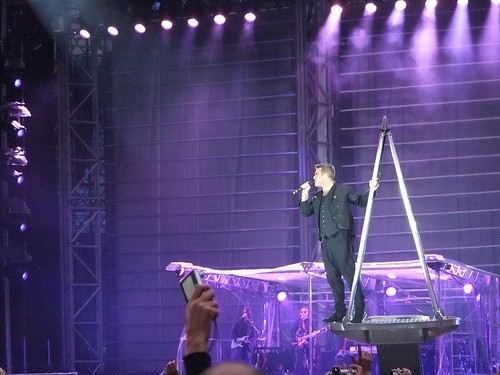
[180,269,202,303]
[329,367,356,375]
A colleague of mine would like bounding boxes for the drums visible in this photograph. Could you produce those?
[444,339,468,370]
[254,347,289,371]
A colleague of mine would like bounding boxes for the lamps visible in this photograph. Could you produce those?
[11,120,27,139]
[9,170,25,185]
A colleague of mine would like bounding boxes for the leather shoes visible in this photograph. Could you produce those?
[322,312,346,322]
[349,316,364,323]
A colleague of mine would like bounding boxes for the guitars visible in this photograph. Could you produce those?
[297,327,327,347]
[231,336,265,349]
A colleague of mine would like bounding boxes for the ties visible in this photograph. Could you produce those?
[302,320,306,330]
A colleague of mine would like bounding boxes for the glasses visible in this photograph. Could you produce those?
[301,311,307,314]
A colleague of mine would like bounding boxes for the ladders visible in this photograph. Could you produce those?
[349,116,444,321]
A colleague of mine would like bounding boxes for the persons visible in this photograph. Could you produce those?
[230,307,256,366]
[289,306,317,374]
[1,281,376,375]
[299,163,381,324]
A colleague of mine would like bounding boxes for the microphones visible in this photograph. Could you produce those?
[292,182,312,194]
